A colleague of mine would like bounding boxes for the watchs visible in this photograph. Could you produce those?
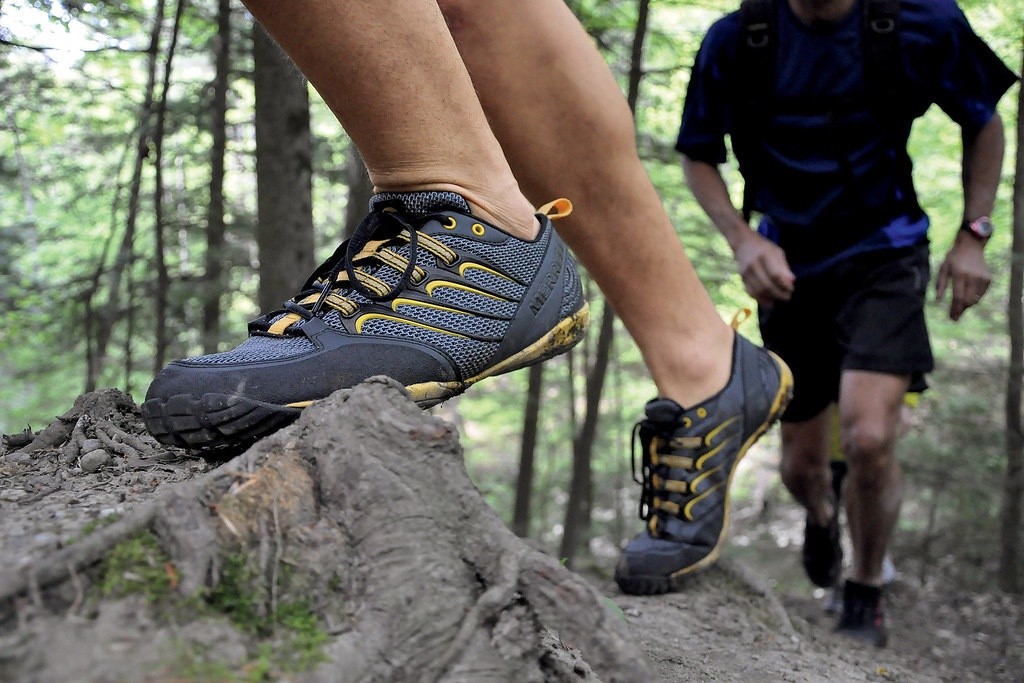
[960,216,993,241]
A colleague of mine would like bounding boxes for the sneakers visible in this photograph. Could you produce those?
[803,459,848,587]
[825,581,843,614]
[140,191,591,450]
[834,570,888,650]
[616,308,792,596]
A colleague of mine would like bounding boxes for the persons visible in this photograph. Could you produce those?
[141,0,794,596]
[828,392,919,498]
[673,1,1020,649]
